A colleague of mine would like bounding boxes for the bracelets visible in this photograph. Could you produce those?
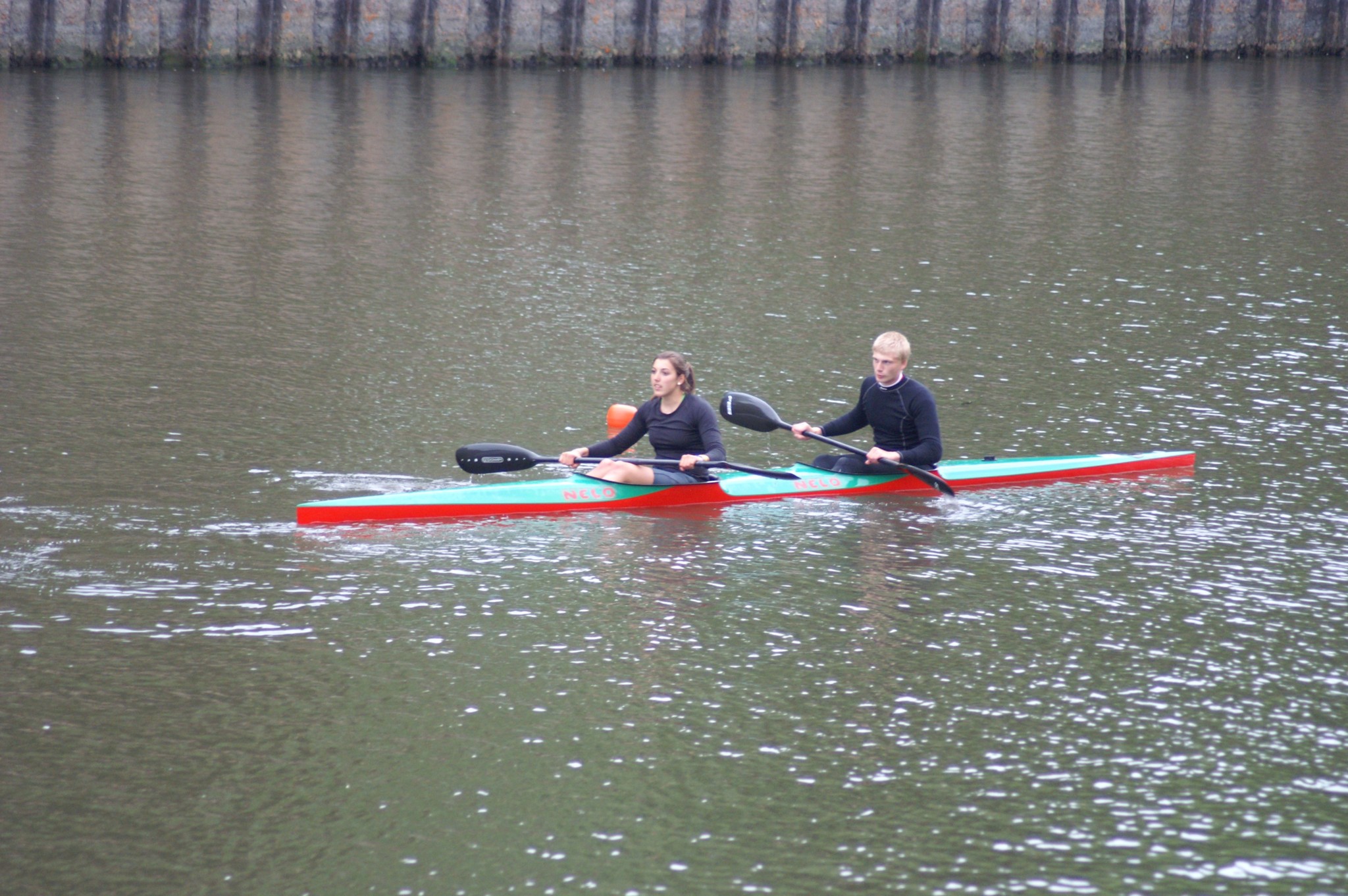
[698,455,705,461]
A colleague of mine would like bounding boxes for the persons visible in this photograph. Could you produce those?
[559,351,726,485]
[791,331,942,474]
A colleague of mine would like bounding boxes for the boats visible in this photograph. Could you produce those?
[297,450,1197,526]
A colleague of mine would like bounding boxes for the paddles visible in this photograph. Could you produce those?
[719,391,955,497]
[455,442,802,480]
